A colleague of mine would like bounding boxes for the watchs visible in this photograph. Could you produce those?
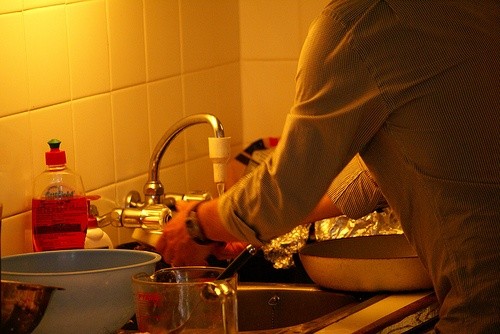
[187,198,226,247]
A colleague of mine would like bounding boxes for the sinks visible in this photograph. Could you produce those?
[236,281,393,334]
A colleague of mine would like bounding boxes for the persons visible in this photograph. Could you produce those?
[150,0,500,334]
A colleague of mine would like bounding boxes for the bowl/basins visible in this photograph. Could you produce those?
[298,235,432,292]
[0,249,162,334]
[0,279,62,334]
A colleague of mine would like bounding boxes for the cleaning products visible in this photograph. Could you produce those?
[84,194,114,249]
[31,138,88,252]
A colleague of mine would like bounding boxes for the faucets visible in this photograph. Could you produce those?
[143,112,233,204]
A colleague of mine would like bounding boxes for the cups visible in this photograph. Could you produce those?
[132,265,238,334]
